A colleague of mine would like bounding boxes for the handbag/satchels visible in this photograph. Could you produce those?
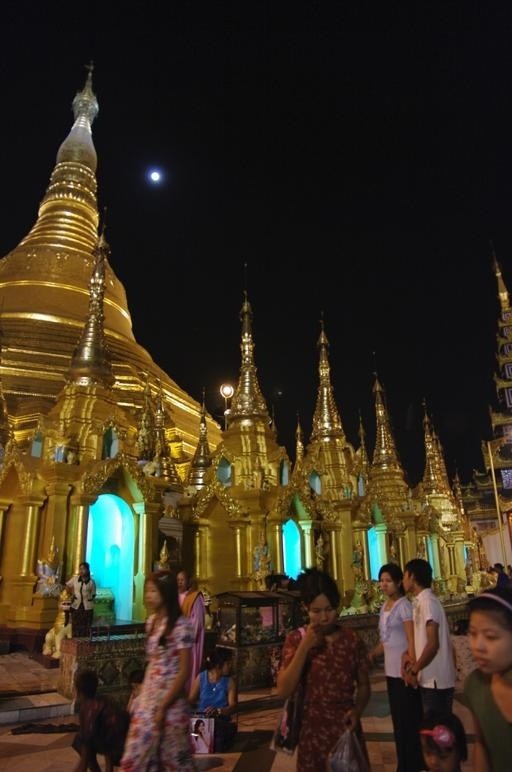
[271,683,298,755]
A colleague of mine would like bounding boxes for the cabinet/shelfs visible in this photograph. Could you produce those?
[212,589,302,690]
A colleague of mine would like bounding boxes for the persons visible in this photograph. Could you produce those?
[63,562,97,636]
[277,558,511,772]
[119,566,238,771]
